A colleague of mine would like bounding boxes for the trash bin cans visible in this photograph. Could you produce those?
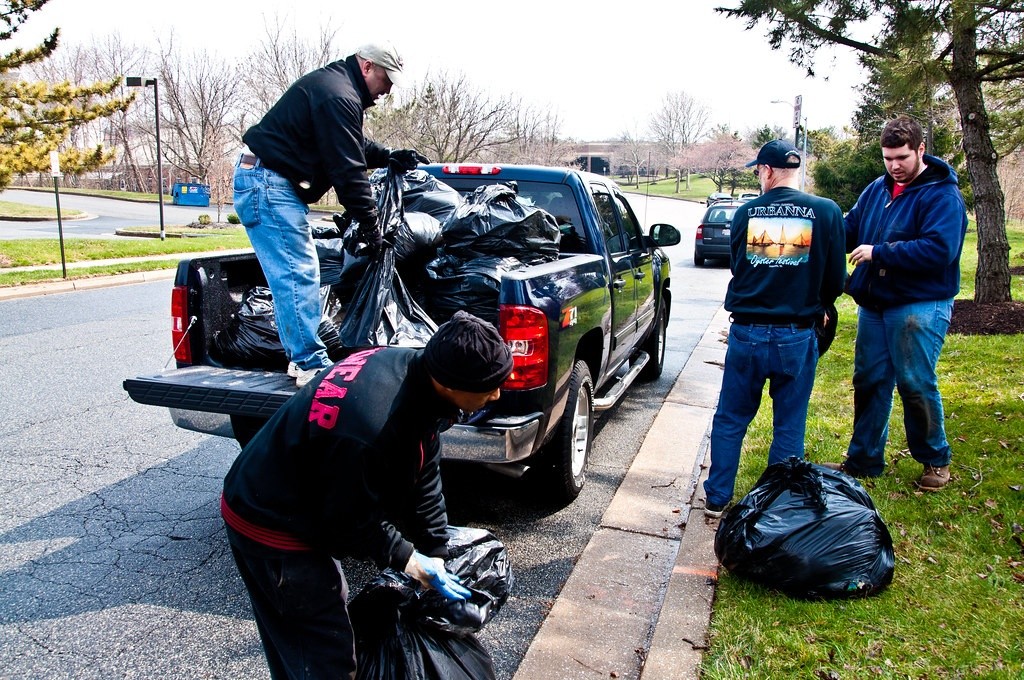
[171,182,211,207]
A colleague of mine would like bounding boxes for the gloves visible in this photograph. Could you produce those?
[388,149,430,174]
[357,226,381,261]
[404,550,472,602]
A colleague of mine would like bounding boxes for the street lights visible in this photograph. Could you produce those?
[125,76,167,240]
[770,99,809,193]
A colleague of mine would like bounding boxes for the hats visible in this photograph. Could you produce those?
[744,138,801,169]
[425,311,514,392]
[358,40,411,88]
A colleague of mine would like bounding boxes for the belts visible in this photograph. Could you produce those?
[239,154,270,169]
[733,318,812,329]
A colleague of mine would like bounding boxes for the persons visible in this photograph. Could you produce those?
[703,139,845,517]
[222,311,513,680]
[823,116,968,491]
[235,42,429,387]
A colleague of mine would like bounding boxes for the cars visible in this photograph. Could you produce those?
[707,193,733,207]
[736,192,760,201]
[694,199,753,266]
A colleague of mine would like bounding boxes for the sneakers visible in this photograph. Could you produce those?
[296,359,334,388]
[287,361,303,378]
[920,463,950,491]
[822,462,868,478]
[704,498,730,518]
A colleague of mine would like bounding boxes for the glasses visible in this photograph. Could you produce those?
[753,165,773,175]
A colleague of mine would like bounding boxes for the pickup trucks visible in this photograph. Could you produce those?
[122,161,682,506]
